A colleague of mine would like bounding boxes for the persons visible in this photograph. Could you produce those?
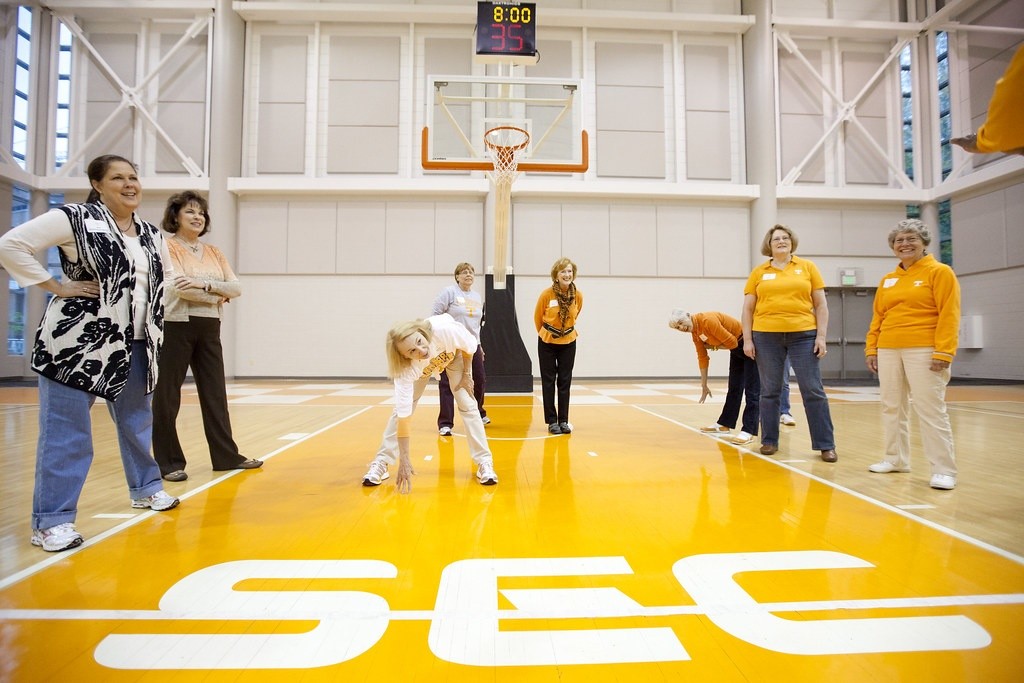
[740,224,839,463]
[362,313,498,494]
[779,354,796,426]
[149,190,264,482]
[533,257,583,435]
[430,262,493,435]
[0,153,183,551]
[667,309,759,445]
[863,219,961,490]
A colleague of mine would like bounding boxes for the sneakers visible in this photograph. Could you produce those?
[559,422,572,434]
[929,473,956,489]
[361,460,390,486]
[131,491,180,511]
[477,464,498,485]
[548,422,562,434]
[439,426,452,436]
[779,414,796,426]
[30,520,85,551]
[482,417,490,424]
[868,459,911,473]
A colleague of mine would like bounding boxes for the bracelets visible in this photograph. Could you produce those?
[203,280,209,292]
[713,345,718,351]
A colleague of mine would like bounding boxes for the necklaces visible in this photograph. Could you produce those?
[124,219,134,233]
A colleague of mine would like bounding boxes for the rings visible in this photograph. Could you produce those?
[825,351,828,353]
[83,287,87,292]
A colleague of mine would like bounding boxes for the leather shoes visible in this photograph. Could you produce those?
[759,443,779,454]
[820,449,837,462]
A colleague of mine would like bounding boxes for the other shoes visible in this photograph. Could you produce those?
[233,459,263,469]
[164,469,188,481]
[701,423,729,433]
[732,430,755,444]
